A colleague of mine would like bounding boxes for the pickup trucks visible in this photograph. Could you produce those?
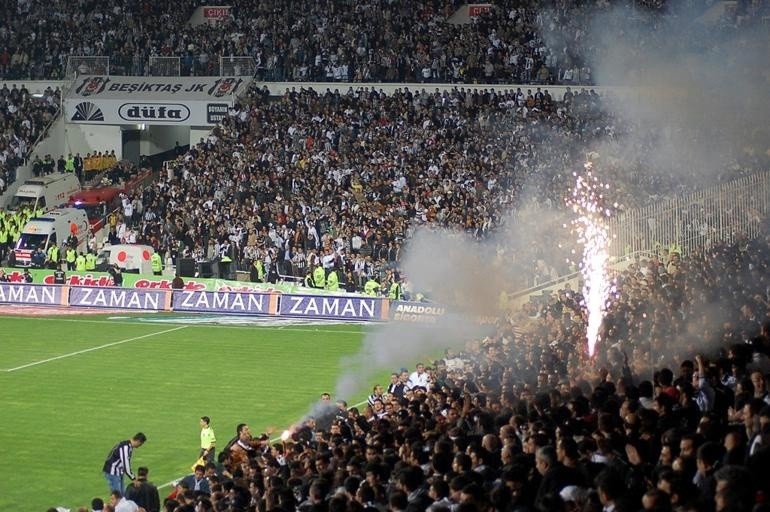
[69,168,152,234]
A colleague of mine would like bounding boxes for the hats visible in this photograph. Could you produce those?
[560,486,582,501]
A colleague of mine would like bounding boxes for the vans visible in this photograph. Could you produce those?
[7,172,81,214]
[11,208,90,268]
